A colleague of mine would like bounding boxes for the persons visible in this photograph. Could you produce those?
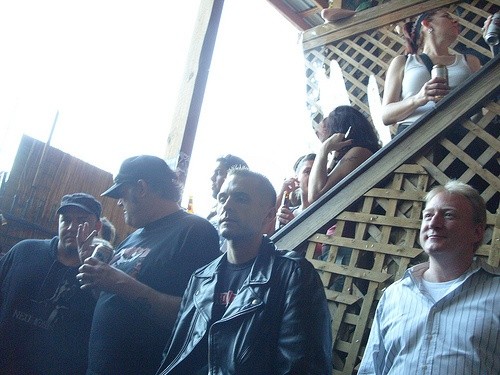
[76,154,219,375]
[382,8,499,214]
[155,168,332,375]
[357,182,500,375]
[0,192,103,375]
[206,105,392,375]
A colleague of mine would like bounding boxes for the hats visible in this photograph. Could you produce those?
[100,155,176,198]
[56,193,101,218]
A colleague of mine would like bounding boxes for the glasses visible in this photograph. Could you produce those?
[217,153,236,164]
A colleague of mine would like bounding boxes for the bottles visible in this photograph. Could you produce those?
[187,196,194,214]
[327,126,355,173]
[277,187,292,232]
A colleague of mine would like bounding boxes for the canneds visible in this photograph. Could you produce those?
[91,243,113,264]
[484,11,500,46]
[431,64,449,103]
